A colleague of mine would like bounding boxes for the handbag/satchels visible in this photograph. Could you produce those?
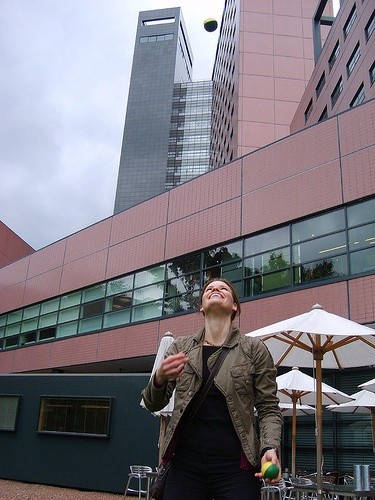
[148,451,173,500]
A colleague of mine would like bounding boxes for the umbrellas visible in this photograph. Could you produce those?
[253,403,323,416]
[358,377,375,394]
[326,389,375,453]
[276,366,355,479]
[242,303,374,500]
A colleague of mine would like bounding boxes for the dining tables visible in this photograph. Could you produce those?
[323,482,375,500]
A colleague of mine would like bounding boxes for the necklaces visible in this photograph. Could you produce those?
[204,337,224,349]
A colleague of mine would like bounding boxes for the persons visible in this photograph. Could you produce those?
[140,277,282,500]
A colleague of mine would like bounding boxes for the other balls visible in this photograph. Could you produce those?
[261,462,278,479]
[204,18,218,32]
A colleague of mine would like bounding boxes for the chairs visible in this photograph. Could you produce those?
[261,478,340,500]
[122,465,153,499]
